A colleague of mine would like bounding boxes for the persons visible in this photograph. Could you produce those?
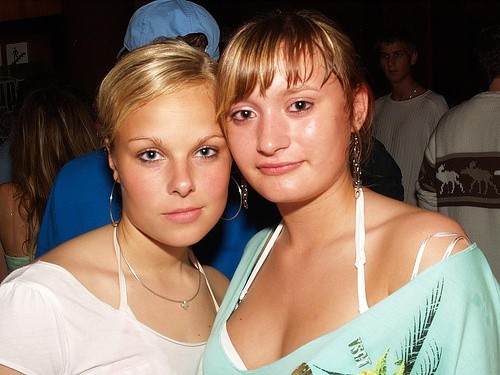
[416,45,499,282]
[0,36,232,375]
[35,0,261,280]
[243,131,405,229]
[371,28,449,207]
[201,15,499,374]
[0,90,107,281]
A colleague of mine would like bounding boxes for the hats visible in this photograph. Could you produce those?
[116,0,219,61]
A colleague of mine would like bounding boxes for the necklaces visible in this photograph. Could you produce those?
[408,82,416,99]
[116,219,201,308]
